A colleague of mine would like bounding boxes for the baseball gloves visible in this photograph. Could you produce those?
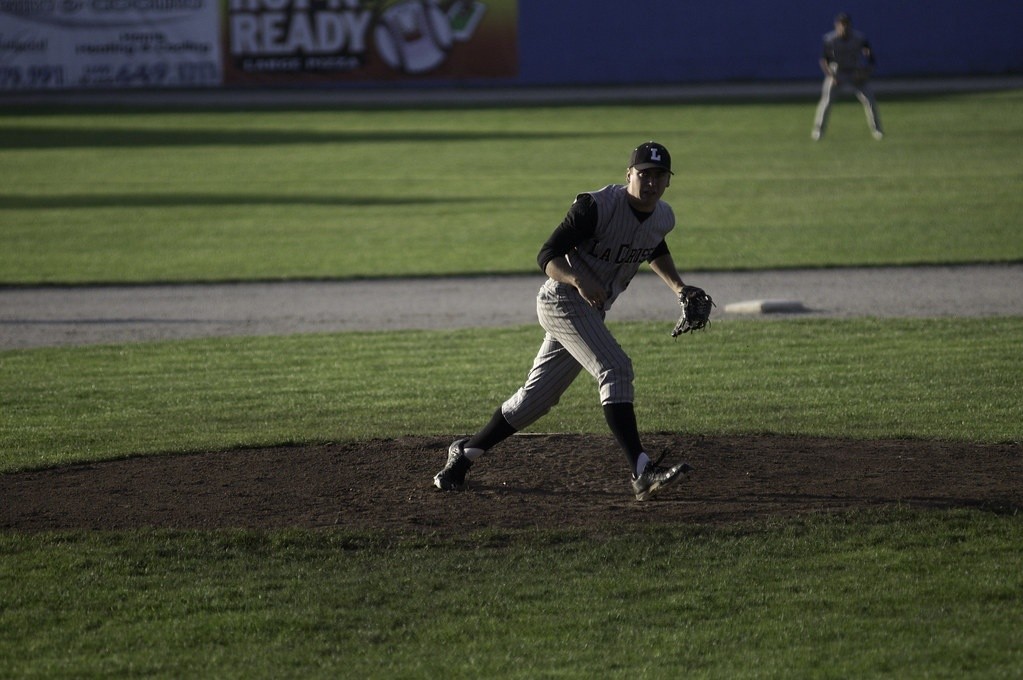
[670,286,715,338]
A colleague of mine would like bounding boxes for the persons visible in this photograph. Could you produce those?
[432,141,713,501]
[812,11,884,142]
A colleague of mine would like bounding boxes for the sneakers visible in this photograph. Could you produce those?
[434,438,470,492]
[630,448,694,501]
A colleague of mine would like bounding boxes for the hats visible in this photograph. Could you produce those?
[629,142,675,177]
